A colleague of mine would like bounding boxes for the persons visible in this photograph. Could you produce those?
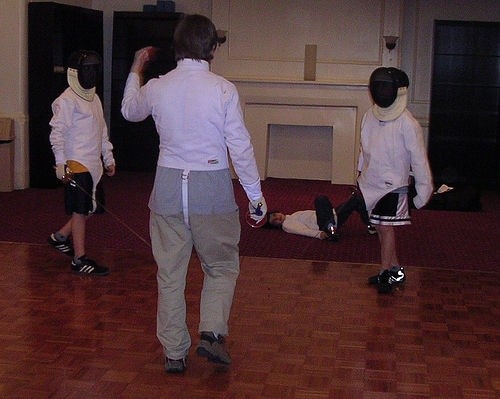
[357,67,433,293]
[262,189,378,241]
[122,14,267,374]
[46,49,116,275]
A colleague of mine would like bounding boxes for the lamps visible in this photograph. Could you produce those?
[216,30,228,47]
[383,36,399,53]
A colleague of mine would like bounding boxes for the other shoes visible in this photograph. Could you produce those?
[327,227,338,240]
[368,225,378,234]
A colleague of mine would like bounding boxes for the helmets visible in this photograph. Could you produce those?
[370,67,410,121]
[67,50,101,101]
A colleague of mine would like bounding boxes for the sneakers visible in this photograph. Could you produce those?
[377,269,393,294]
[164,357,186,372]
[196,332,232,364]
[368,266,405,285]
[71,255,109,275]
[47,233,75,257]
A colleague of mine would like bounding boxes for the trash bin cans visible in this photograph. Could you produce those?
[0,117,15,191]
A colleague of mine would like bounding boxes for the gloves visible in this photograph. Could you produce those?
[249,197,267,221]
[56,164,72,183]
